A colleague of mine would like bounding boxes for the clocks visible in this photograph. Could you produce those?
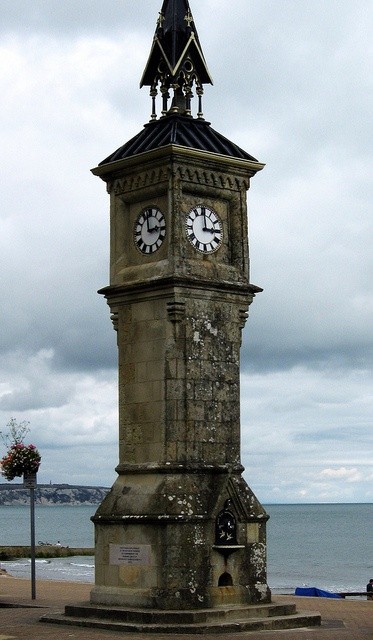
[184,203,224,255]
[133,207,167,254]
